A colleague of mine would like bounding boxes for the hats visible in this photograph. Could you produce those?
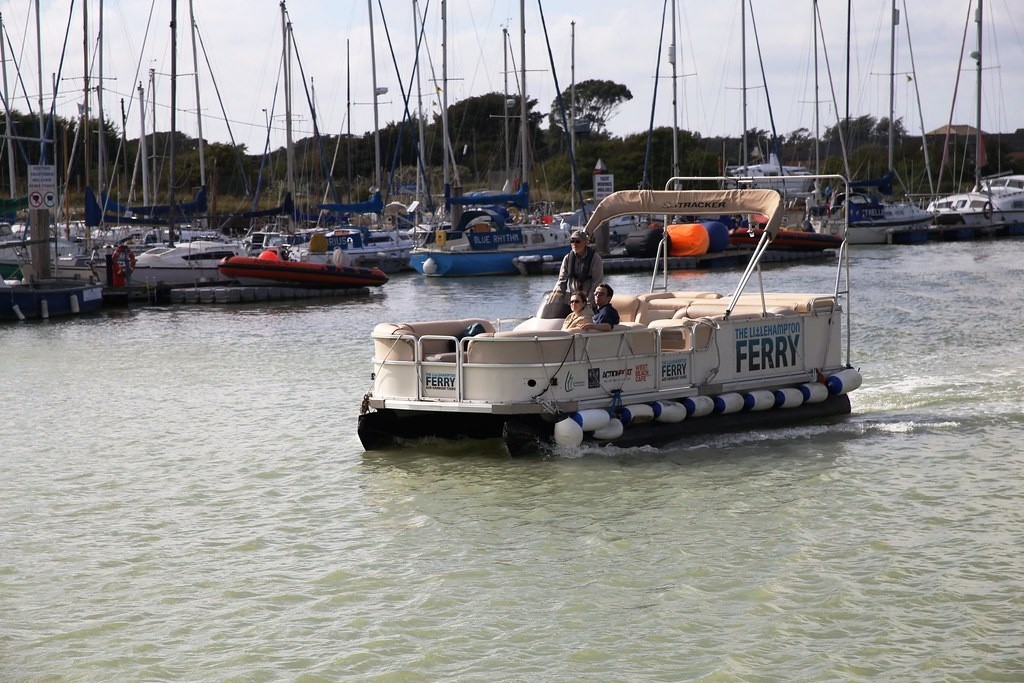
[570,231,586,240]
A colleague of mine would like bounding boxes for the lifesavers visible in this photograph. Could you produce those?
[983,201,993,219]
[506,206,519,222]
[113,245,136,278]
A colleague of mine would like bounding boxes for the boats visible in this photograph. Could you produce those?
[356,173,864,460]
[219,255,390,291]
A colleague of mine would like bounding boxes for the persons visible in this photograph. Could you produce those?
[553,231,603,313]
[825,186,832,203]
[580,284,620,331]
[561,291,593,333]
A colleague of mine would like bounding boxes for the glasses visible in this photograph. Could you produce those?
[569,240,583,244]
[569,300,584,303]
[594,292,608,296]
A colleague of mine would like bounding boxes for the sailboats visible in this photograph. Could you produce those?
[0,1,1024,320]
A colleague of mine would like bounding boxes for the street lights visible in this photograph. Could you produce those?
[262,108,273,199]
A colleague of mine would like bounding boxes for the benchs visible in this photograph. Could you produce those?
[372,292,841,364]
